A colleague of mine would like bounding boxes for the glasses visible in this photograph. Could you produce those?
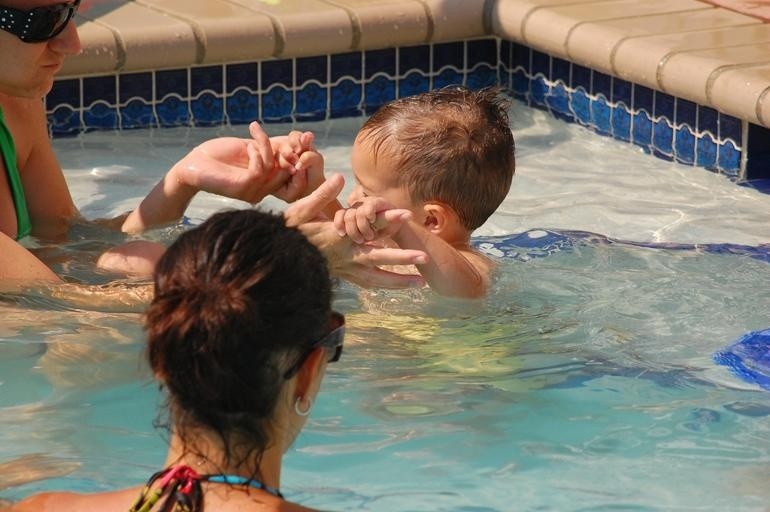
[1,0,82,45]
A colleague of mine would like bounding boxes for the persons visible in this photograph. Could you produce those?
[0,1,430,416]
[263,78,518,426]
[1,205,346,512]
